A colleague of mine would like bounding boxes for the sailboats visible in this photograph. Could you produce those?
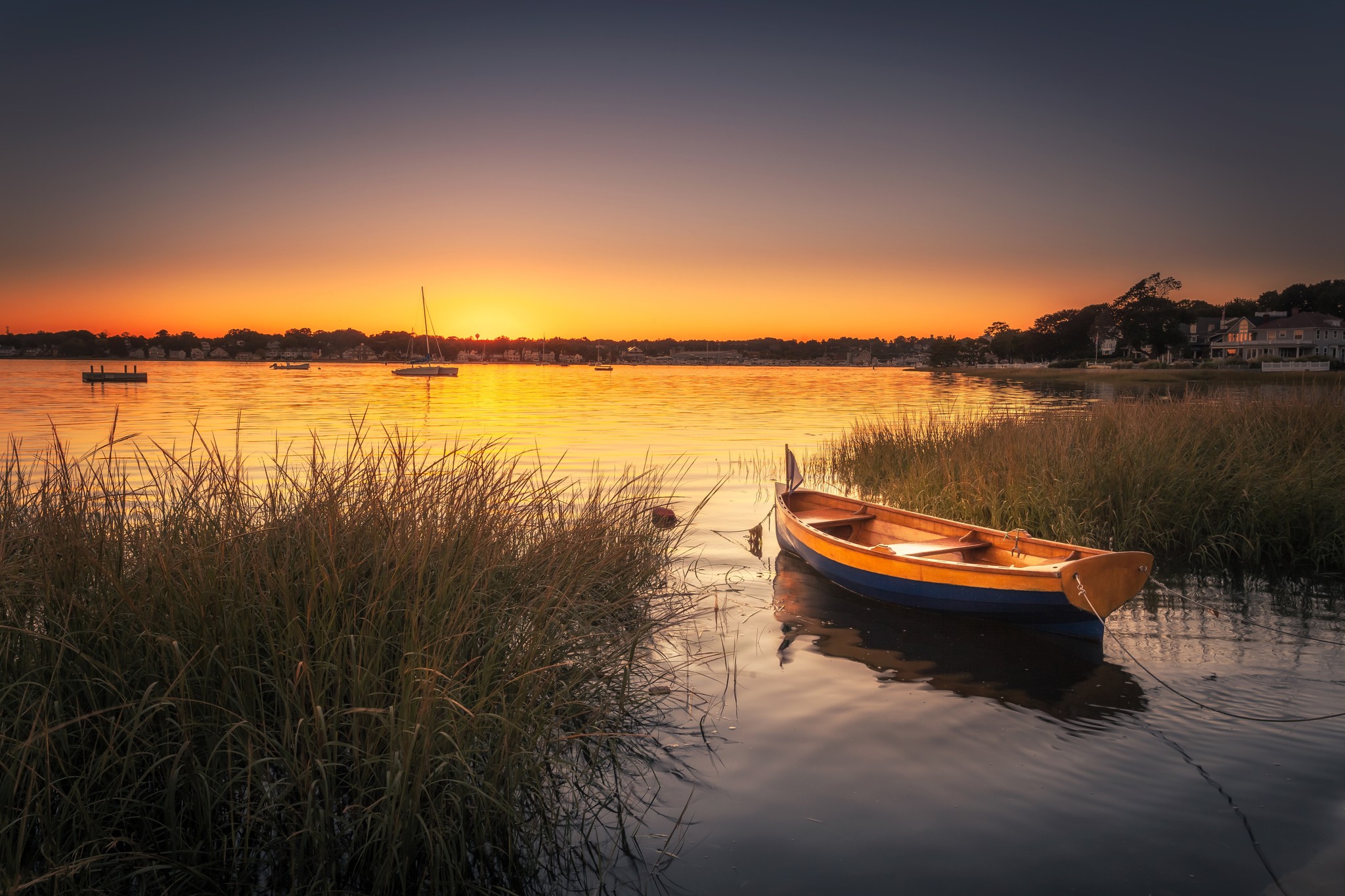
[629,341,638,366]
[596,340,602,365]
[391,284,459,377]
[702,342,823,372]
[594,349,614,371]
[480,342,489,365]
[404,326,427,364]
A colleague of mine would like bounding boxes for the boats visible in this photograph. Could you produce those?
[379,362,389,364]
[535,363,541,366]
[269,362,310,369]
[561,363,568,366]
[542,362,550,365]
[773,443,1156,644]
[588,360,595,366]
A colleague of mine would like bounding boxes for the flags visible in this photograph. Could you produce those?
[1096,336,1098,347]
[1222,310,1225,321]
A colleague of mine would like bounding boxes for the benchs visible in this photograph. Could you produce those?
[870,535,992,563]
[1022,556,1078,572]
[793,507,876,543]
